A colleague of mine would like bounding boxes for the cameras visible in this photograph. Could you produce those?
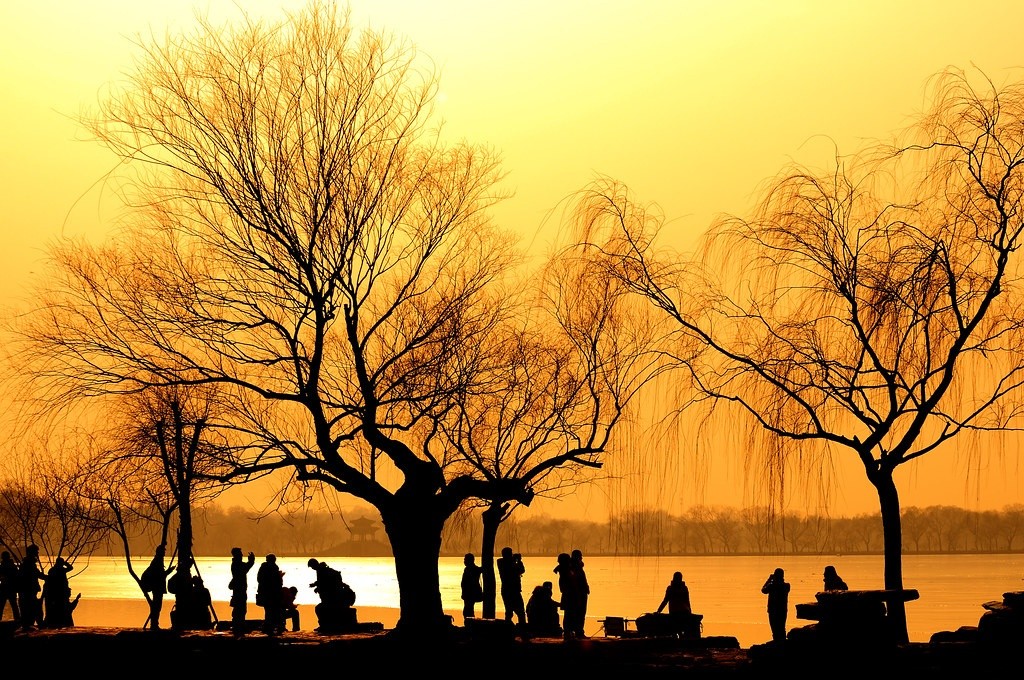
[513,554,519,559]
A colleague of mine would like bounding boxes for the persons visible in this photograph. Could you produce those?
[653,571,692,615]
[257,553,286,634]
[314,577,348,636]
[823,566,848,591]
[0,545,82,636]
[308,558,343,636]
[497,547,529,641]
[149,545,212,634]
[282,586,300,632]
[761,568,790,644]
[526,550,590,644]
[461,553,483,619]
[230,547,255,636]
